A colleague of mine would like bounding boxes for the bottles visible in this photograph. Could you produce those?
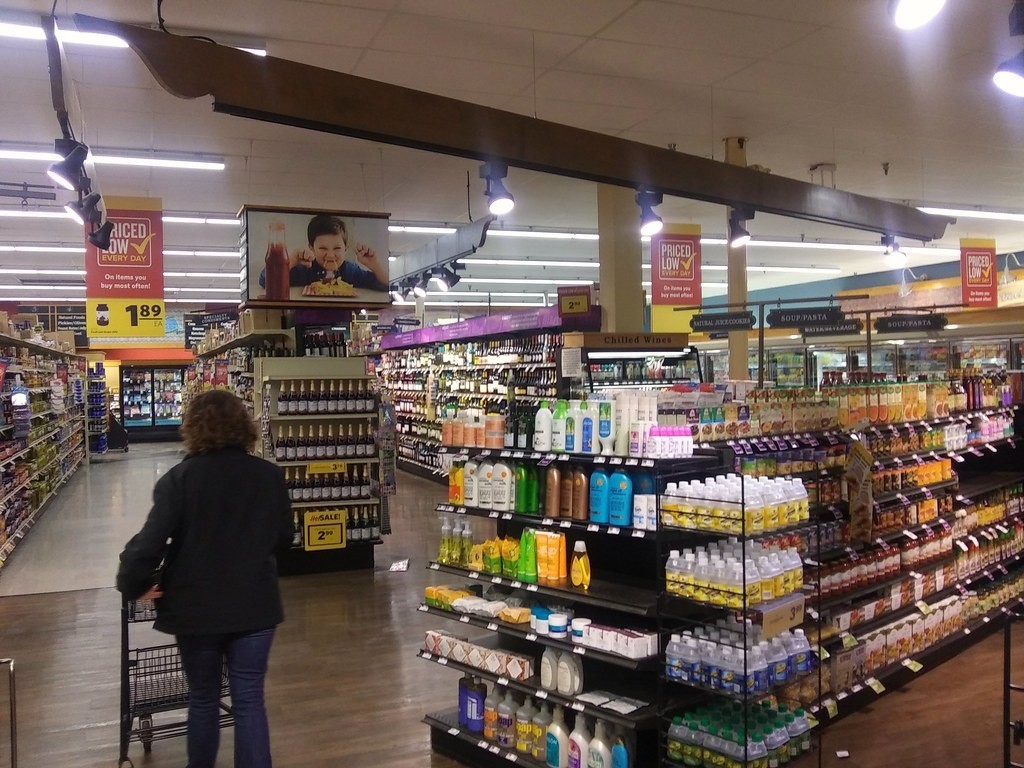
[662,617,815,767]
[299,331,347,357]
[291,506,382,548]
[272,422,376,461]
[283,465,373,503]
[277,381,375,413]
[660,473,811,608]
[819,370,929,389]
[945,368,1012,413]
[248,346,293,374]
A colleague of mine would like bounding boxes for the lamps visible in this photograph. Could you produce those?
[728,207,755,250]
[64,191,102,225]
[993,48,1024,98]
[87,221,114,251]
[879,236,908,270]
[634,189,665,239]
[887,1,947,31]
[479,159,515,217]
[45,145,87,193]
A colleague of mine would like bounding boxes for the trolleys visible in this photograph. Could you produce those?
[119,590,235,768]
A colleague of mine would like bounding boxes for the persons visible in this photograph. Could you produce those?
[259,215,388,293]
[116,390,296,768]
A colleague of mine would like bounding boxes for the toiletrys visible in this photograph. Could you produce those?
[420,384,698,768]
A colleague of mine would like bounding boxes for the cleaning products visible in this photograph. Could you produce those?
[0,318,110,571]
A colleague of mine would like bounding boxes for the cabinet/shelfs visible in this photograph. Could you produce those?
[0,297,1024,766]
